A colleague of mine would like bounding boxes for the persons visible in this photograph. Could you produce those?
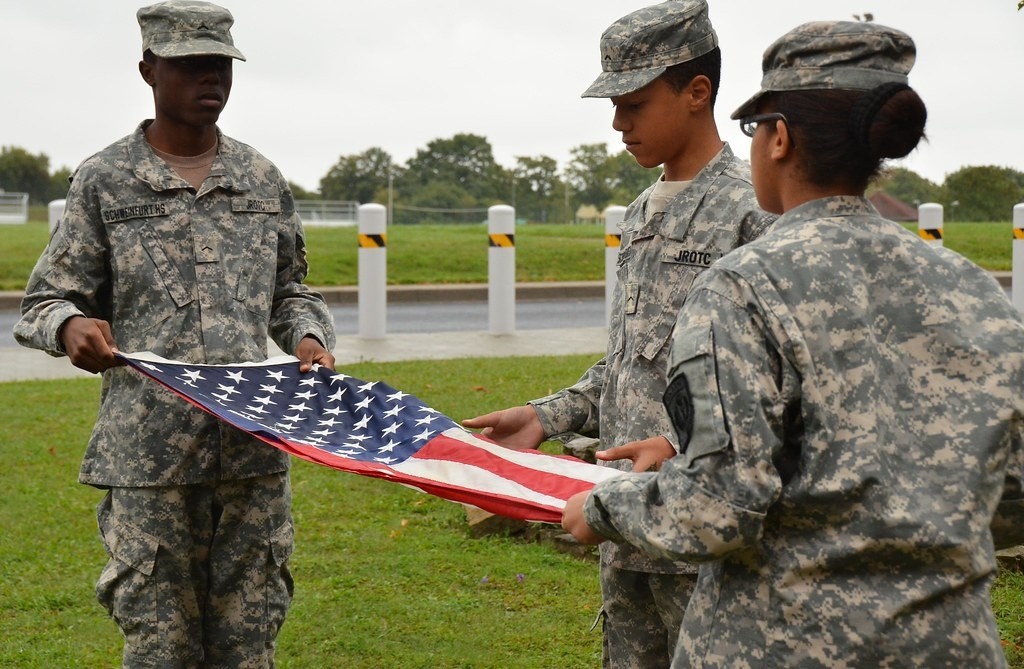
[558,17,1024,667]
[455,0,784,664]
[7,0,338,667]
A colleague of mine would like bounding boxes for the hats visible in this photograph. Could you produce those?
[580,0,721,102]
[729,17,923,119]
[133,2,249,64]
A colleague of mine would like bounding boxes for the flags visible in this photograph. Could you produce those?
[105,335,641,532]
